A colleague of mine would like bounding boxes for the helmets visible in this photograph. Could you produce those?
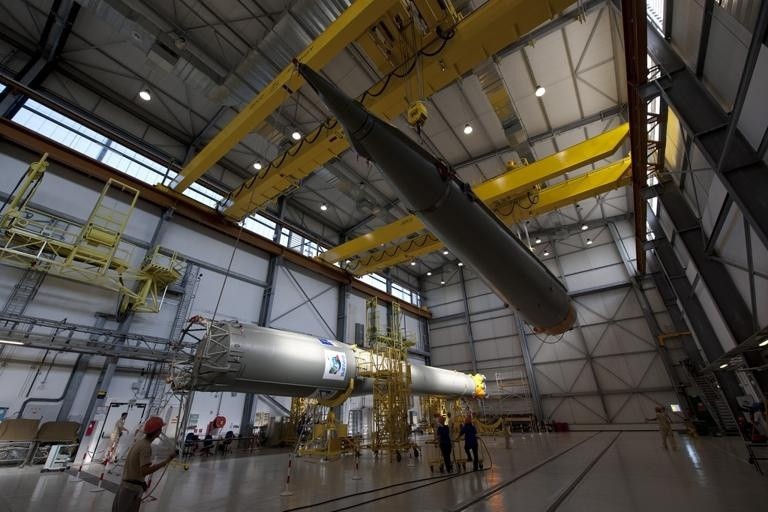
[142,416,167,434]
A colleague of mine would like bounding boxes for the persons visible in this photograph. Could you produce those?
[456,418,478,471]
[102,413,129,464]
[646,406,677,451]
[437,416,452,472]
[112,417,177,512]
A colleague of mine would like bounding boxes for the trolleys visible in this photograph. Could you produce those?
[450,437,486,471]
[423,438,461,475]
